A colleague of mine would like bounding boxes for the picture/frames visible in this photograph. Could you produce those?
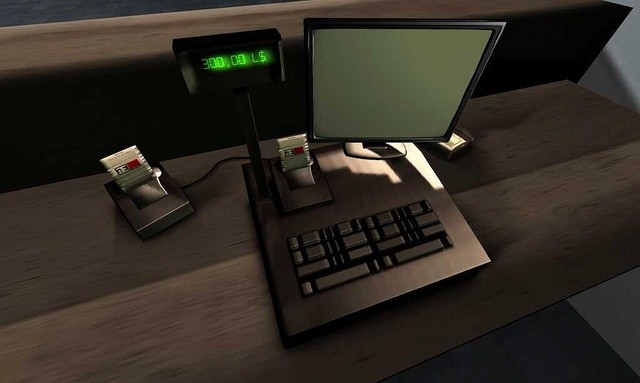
[1,0,639,382]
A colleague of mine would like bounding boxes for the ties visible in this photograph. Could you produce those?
[302,17,508,160]
